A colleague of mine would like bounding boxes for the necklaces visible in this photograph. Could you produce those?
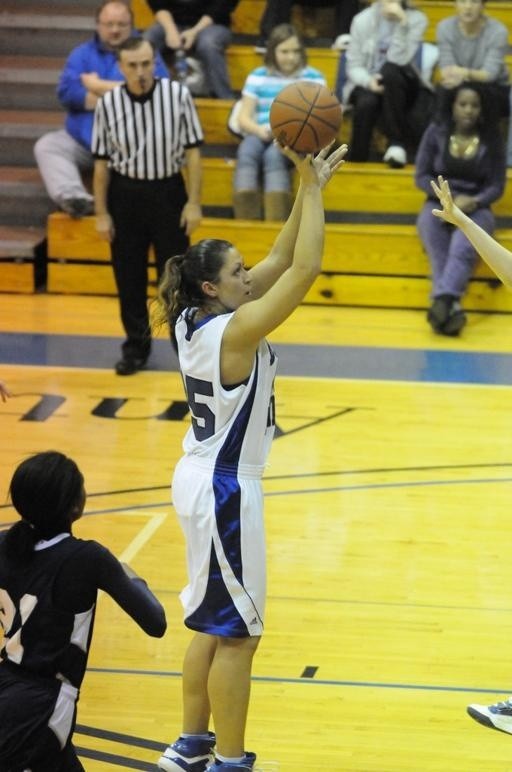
[449,134,480,156]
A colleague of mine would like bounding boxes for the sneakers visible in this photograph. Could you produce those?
[426,297,449,328]
[384,146,408,167]
[444,310,466,333]
[467,699,512,736]
[116,354,147,374]
[203,752,261,772]
[64,193,96,216]
[158,732,215,771]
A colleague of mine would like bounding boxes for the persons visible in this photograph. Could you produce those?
[145,0,240,103]
[413,84,506,336]
[433,1,512,127]
[0,452,167,772]
[233,23,330,223]
[346,0,428,168]
[430,175,512,735]
[89,38,208,377]
[254,1,357,54]
[32,1,171,216]
[158,136,350,772]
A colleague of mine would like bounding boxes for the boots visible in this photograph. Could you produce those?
[231,190,262,220]
[264,192,290,223]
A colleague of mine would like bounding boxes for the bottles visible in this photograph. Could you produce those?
[175,50,186,80]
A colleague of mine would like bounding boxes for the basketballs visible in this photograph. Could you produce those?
[269,81,341,156]
